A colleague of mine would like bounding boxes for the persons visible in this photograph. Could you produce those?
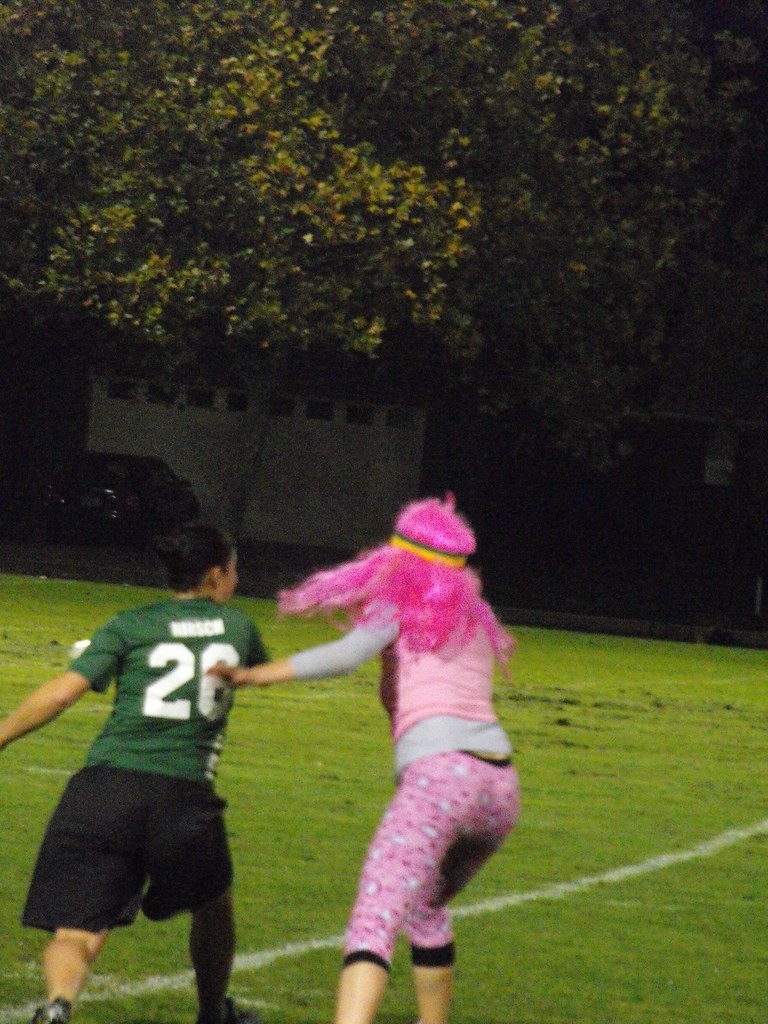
[208,490,522,1024]
[0,524,272,1024]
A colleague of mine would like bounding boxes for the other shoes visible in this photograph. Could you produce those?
[32,997,72,1024]
[197,998,260,1024]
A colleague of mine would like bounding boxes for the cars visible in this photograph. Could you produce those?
[37,453,203,552]
